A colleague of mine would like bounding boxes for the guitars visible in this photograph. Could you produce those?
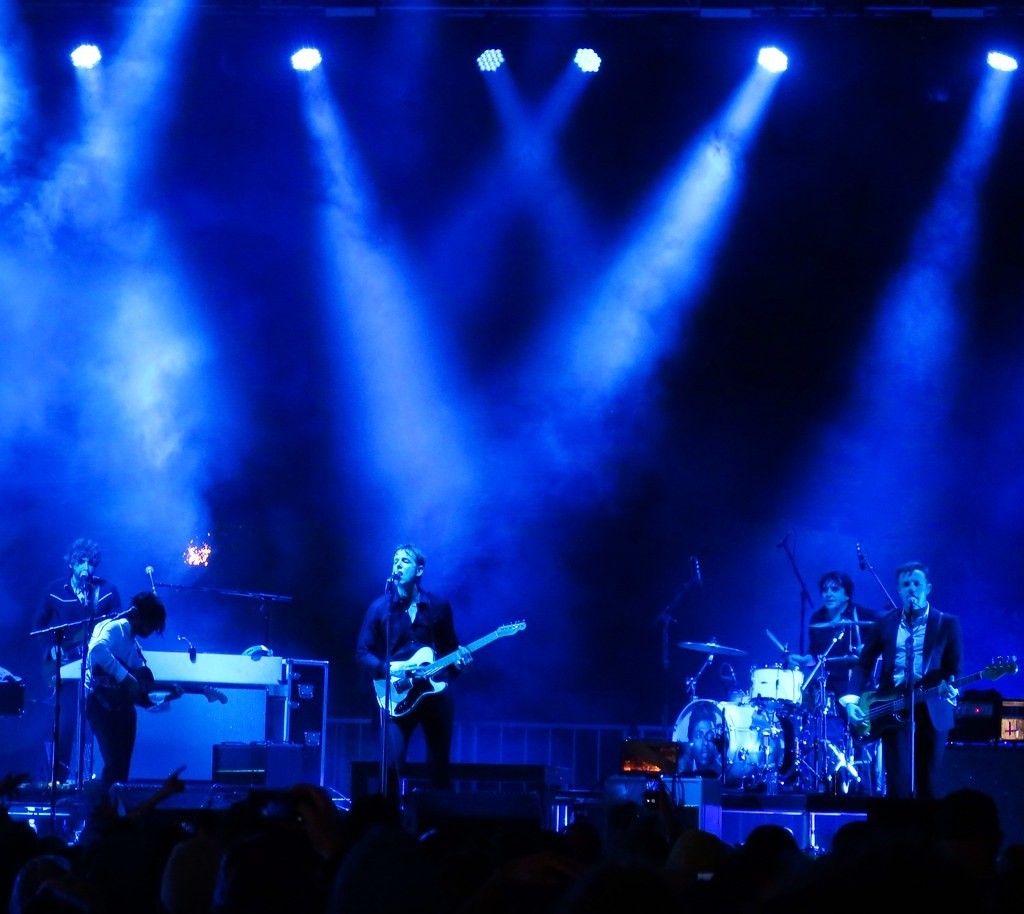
[91,655,226,712]
[848,653,1020,745]
[373,618,527,717]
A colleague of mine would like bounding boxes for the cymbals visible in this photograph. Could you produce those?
[826,655,860,664]
[810,619,874,628]
[678,640,748,659]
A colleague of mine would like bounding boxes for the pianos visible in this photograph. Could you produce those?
[59,633,288,806]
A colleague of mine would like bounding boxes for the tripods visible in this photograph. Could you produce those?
[781,626,877,798]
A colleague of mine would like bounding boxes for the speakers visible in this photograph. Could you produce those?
[211,744,307,789]
[604,775,720,864]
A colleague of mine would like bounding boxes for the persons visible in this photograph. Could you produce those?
[0,764,1024,914]
[36,539,123,785]
[837,561,964,799]
[356,544,473,794]
[85,591,184,801]
[788,571,883,700]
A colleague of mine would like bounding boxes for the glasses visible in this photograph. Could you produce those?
[76,559,95,567]
[899,580,927,587]
[821,587,845,592]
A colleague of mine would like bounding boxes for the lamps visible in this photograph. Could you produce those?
[619,737,684,776]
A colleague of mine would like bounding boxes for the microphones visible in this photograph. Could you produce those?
[775,531,792,548]
[387,571,401,582]
[694,558,704,591]
[80,571,89,589]
[907,597,917,604]
[145,566,158,598]
[856,543,865,570]
[728,665,736,683]
[111,606,138,622]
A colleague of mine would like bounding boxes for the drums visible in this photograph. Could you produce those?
[748,664,805,708]
[671,696,783,784]
